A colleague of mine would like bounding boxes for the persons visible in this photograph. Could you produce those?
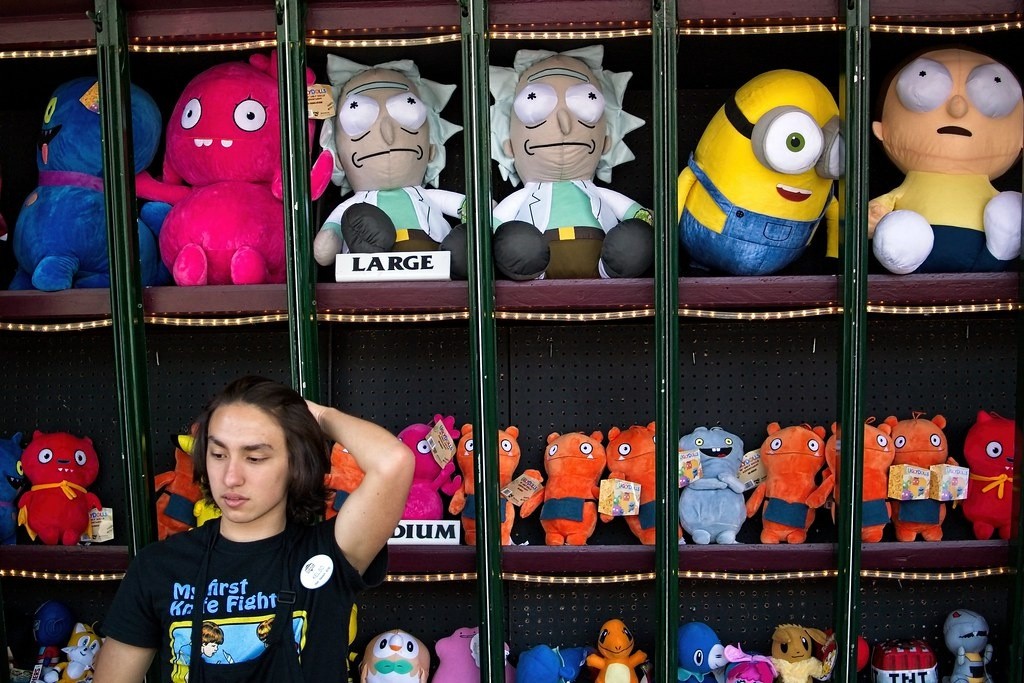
[92,379,415,683]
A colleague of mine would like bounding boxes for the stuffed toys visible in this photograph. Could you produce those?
[358,619,651,683]
[867,47,1024,276]
[9,77,174,292]
[134,52,334,286]
[677,69,841,277]
[153,421,222,540]
[322,409,1016,547]
[871,609,994,683]
[677,622,838,683]
[313,55,466,282]
[0,429,102,546]
[32,600,102,683]
[488,45,653,281]
